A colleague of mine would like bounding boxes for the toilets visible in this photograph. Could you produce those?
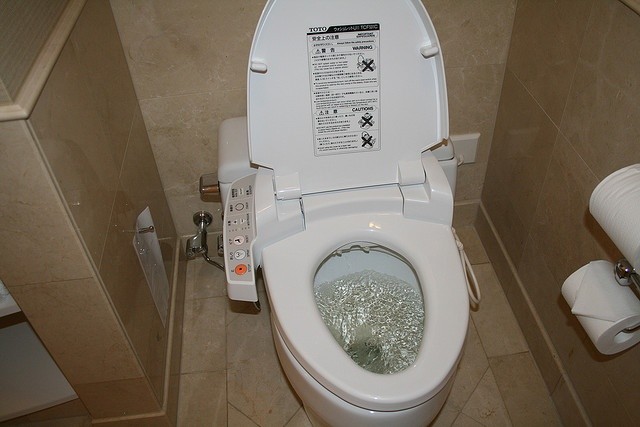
[215,1,471,426]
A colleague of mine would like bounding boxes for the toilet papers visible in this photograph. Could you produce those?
[589,162,639,276]
[559,260,636,357]
